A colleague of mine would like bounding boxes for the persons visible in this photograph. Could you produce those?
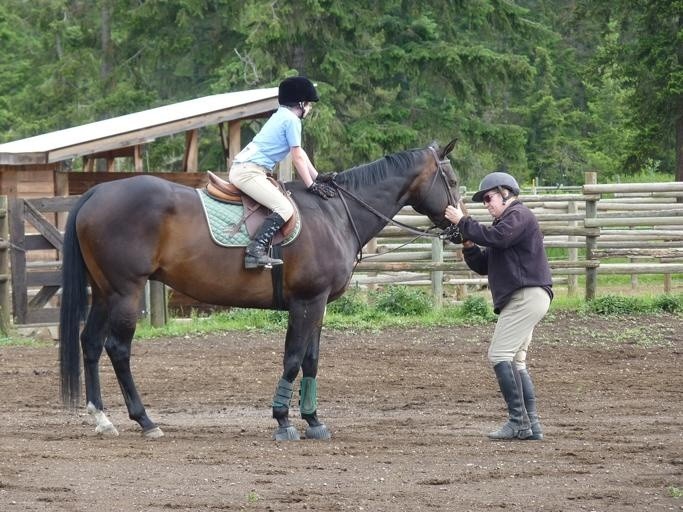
[443,170,555,441]
[226,76,337,270]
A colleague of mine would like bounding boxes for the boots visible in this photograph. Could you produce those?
[487,360,532,441]
[516,367,543,440]
[243,212,286,270]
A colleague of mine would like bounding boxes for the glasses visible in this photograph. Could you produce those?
[481,192,499,204]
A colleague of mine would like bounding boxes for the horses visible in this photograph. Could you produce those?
[57,137,472,444]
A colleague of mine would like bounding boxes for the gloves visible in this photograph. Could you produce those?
[314,172,338,185]
[306,183,335,200]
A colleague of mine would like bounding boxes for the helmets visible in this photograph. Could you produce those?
[471,171,520,202]
[277,77,319,105]
